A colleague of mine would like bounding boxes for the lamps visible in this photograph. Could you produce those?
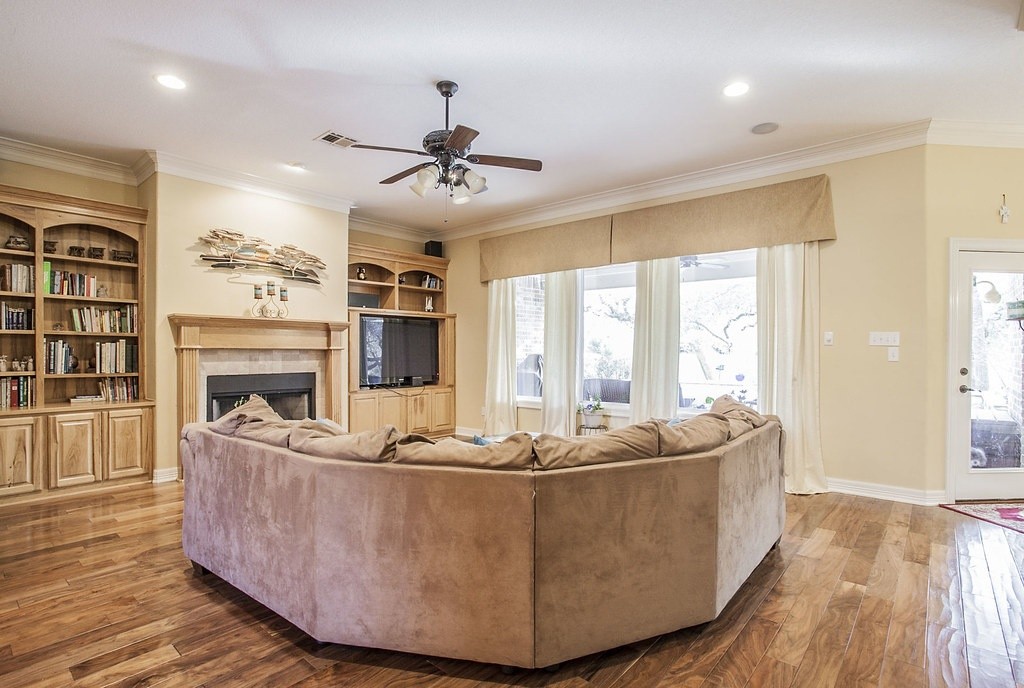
[1005,299,1024,331]
[973,275,1000,303]
[409,147,486,197]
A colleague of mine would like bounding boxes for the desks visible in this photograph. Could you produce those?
[576,425,608,435]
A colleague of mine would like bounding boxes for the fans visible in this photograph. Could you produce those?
[351,80,542,184]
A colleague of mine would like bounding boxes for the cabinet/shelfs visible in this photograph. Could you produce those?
[348,241,457,440]
[0,184,156,507]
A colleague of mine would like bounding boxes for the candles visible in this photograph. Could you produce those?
[252,281,289,319]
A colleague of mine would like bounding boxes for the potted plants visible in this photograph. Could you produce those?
[578,394,604,428]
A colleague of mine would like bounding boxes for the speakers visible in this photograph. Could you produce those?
[425,240,443,258]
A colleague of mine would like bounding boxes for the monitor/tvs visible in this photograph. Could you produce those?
[359,313,440,388]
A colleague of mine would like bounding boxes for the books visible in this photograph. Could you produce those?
[421,273,444,290]
[0,301,35,330]
[0,262,36,292]
[71,302,138,333]
[44,261,97,298]
[69,376,139,403]
[44,334,138,375]
[0,376,36,409]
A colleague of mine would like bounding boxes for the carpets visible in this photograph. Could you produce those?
[939,502,1024,533]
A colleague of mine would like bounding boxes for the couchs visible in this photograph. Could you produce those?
[180,394,786,668]
[583,376,694,407]
